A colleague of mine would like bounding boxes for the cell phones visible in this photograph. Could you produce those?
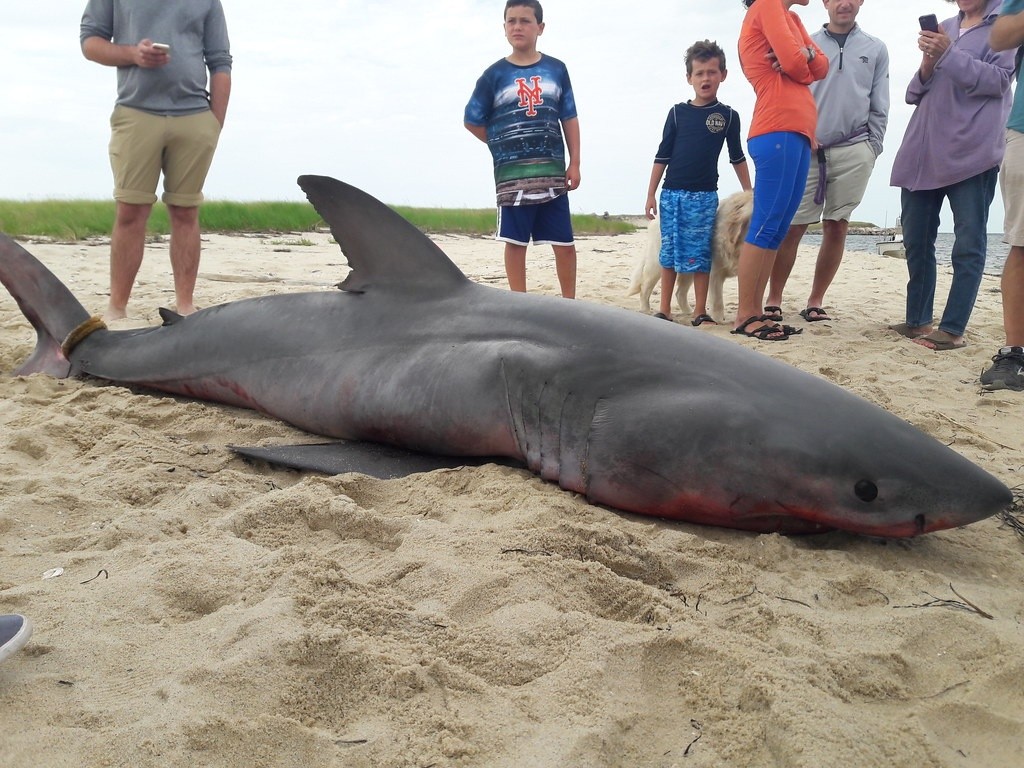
[152,43,169,50]
[919,14,939,33]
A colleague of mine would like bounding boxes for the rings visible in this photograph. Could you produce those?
[924,43,929,49]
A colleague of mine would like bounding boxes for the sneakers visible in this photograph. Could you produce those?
[980,345,1024,392]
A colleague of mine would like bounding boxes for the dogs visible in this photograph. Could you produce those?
[623,188,755,324]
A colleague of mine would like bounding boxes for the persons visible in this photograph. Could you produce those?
[735,1,889,340]
[892,0,1024,393]
[463,0,582,299]
[79,0,233,318]
[643,40,753,330]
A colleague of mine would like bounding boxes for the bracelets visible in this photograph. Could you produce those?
[808,48,814,61]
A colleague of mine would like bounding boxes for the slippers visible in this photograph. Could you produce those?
[690,315,718,327]
[912,330,967,351]
[798,307,831,323]
[879,322,933,339]
[653,312,674,322]
[763,306,783,322]
[730,314,804,340]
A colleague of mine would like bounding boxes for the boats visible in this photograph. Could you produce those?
[875,211,906,260]
